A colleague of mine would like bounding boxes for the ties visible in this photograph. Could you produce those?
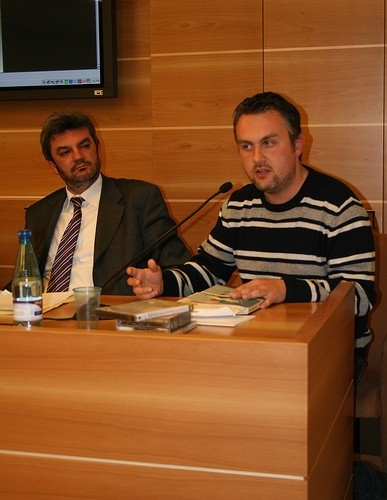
[46,197,85,292]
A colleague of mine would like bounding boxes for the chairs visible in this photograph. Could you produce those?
[352,234,387,474]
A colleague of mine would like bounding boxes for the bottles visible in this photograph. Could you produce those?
[13,229,43,329]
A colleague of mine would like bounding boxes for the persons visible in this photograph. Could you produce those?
[3,110,192,297]
[126,91,387,500]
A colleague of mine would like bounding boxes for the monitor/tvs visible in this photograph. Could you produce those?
[0,0,119,100]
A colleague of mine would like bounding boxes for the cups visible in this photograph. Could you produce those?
[73,287,101,331]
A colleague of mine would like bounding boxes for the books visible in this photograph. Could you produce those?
[171,321,197,336]
[95,299,194,322]
[115,313,190,330]
[178,284,266,314]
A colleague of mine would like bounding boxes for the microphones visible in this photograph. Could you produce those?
[73,182,233,320]
